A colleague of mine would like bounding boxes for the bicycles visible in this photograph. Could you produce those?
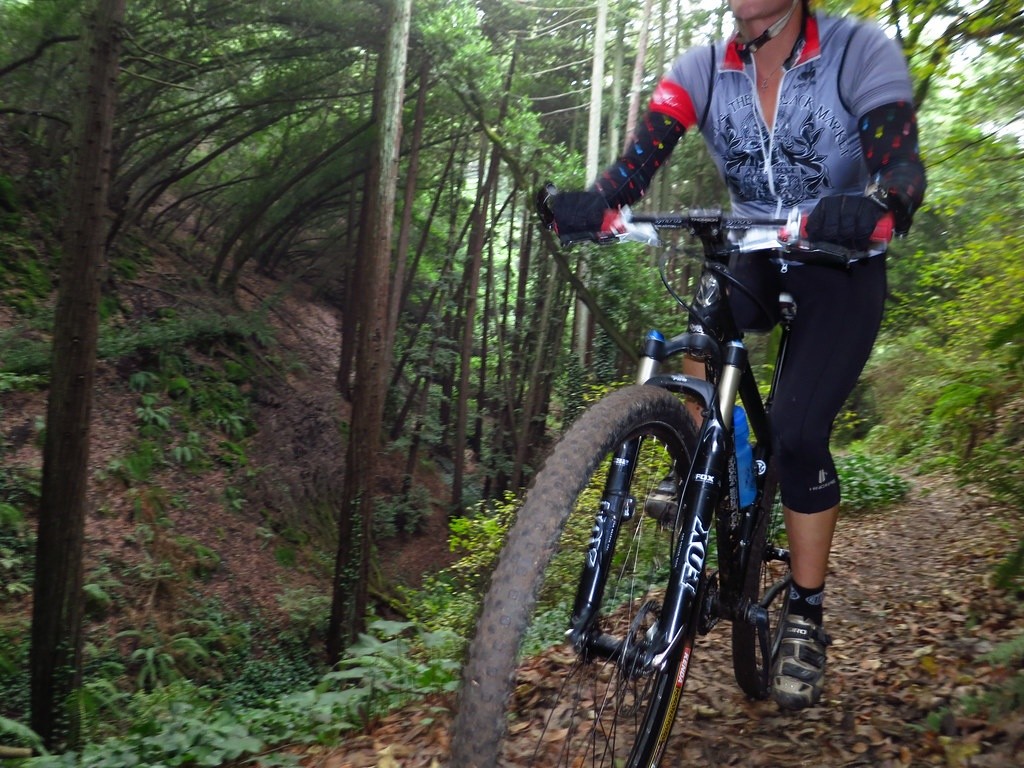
[447,175,896,768]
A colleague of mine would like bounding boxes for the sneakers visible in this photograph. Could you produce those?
[766,614,833,713]
[645,469,681,521]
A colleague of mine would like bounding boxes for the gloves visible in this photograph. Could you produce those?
[551,190,625,246]
[799,186,911,253]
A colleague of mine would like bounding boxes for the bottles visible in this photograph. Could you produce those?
[733,404,758,508]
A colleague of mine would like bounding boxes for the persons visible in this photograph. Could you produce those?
[547,0,926,710]
